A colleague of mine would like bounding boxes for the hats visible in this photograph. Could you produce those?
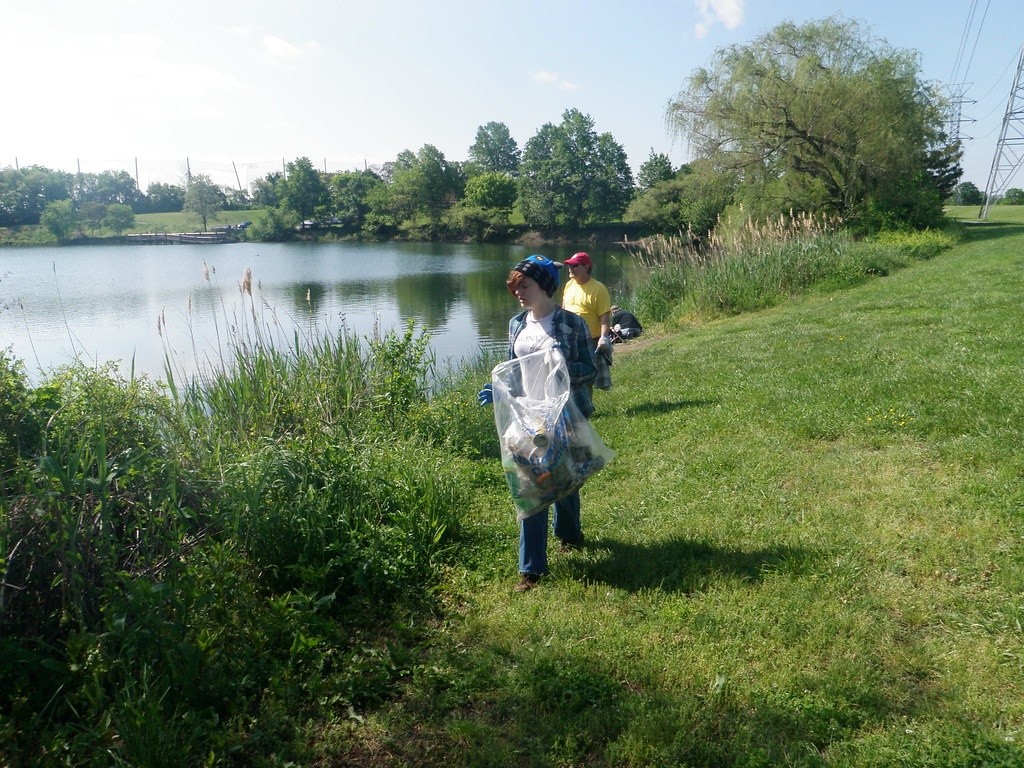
[514,255,563,297]
[565,253,591,265]
[611,305,619,310]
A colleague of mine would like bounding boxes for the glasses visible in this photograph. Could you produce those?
[569,264,580,267]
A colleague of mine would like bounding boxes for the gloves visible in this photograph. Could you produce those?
[552,341,564,349]
[478,384,493,407]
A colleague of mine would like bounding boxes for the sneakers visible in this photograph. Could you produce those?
[515,574,541,591]
[556,542,585,553]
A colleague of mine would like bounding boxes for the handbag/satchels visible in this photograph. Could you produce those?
[492,346,618,522]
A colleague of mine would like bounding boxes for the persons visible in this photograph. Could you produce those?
[478,254,599,592]
[606,305,643,346]
[560,253,615,392]
